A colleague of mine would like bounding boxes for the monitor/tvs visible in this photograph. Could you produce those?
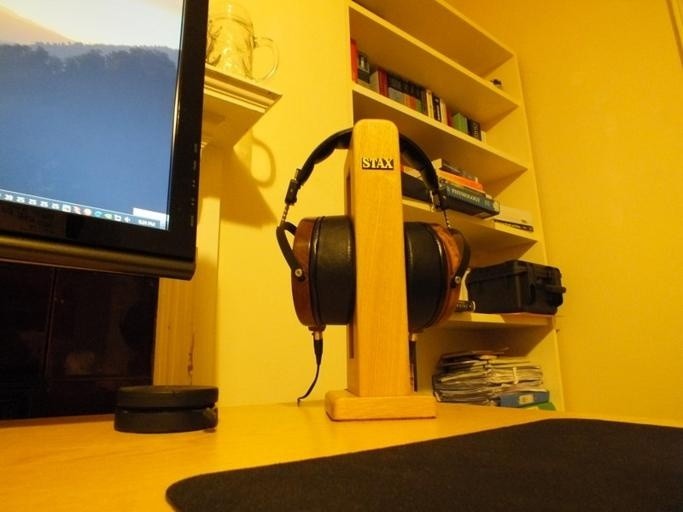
[0,0,209,281]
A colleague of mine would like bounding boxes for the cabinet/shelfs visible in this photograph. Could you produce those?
[213,0,566,414]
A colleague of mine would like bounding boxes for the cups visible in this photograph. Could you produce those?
[204,5,279,83]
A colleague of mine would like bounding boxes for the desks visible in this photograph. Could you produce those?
[0,398,683,512]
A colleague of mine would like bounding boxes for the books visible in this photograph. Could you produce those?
[350,35,491,147]
[432,351,542,405]
[400,151,536,234]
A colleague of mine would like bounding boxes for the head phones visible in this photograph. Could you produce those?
[276,127,471,332]
